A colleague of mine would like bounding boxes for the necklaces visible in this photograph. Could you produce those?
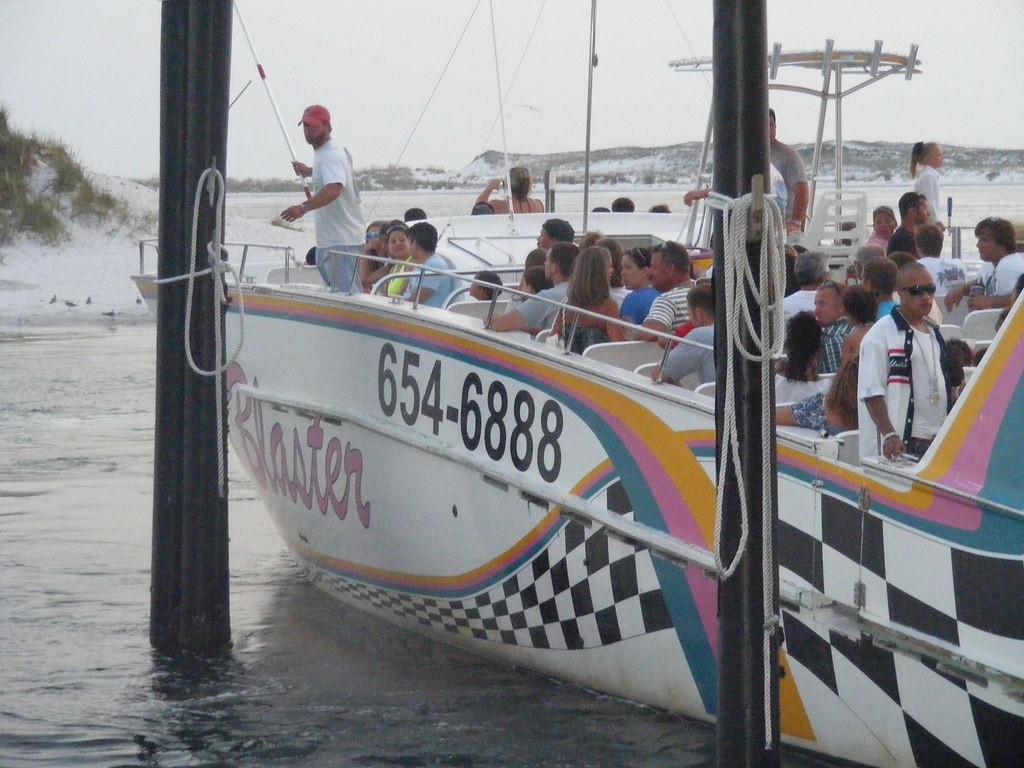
[899,309,939,406]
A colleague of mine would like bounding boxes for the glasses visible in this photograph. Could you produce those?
[661,241,670,266]
[365,231,381,240]
[903,283,936,296]
[626,245,648,268]
[991,217,1001,237]
[825,279,843,296]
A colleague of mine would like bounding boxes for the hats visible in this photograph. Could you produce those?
[298,104,330,126]
[873,205,895,221]
[504,167,532,191]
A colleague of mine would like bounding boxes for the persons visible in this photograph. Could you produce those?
[485,191,1024,437]
[475,167,544,214]
[364,208,466,309]
[909,141,945,232]
[280,105,364,294]
[470,271,504,301]
[857,261,947,461]
[684,107,809,236]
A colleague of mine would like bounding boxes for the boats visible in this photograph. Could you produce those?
[224,0,1022,768]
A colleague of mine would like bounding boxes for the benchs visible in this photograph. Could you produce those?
[447,300,509,319]
[697,372,835,400]
[636,363,703,393]
[963,308,1003,342]
[934,295,972,328]
[582,340,668,372]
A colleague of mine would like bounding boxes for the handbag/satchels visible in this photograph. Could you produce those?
[544,295,572,350]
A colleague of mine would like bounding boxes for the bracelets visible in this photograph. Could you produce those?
[790,220,802,227]
[883,432,898,440]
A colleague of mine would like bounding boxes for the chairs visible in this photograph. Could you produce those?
[794,190,869,274]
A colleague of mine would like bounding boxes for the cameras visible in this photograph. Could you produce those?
[498,180,506,187]
